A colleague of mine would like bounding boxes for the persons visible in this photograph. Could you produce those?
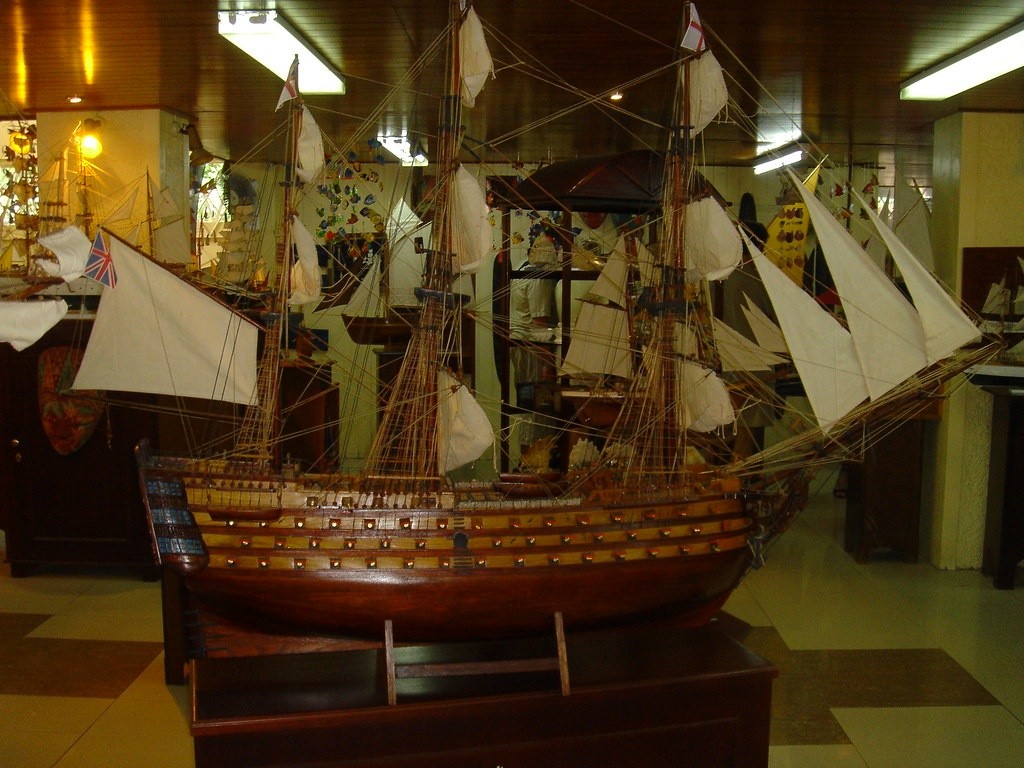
[556,242,607,386]
[508,224,558,459]
[723,220,778,485]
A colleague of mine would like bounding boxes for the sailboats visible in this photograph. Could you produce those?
[22,2,1013,647]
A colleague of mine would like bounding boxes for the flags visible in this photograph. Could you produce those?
[83,227,117,290]
[275,58,297,112]
[681,3,705,52]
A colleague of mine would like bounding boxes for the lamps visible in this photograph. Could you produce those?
[79,118,103,159]
[377,136,430,166]
[179,122,214,166]
[752,143,811,175]
[899,22,1024,102]
[218,9,347,95]
